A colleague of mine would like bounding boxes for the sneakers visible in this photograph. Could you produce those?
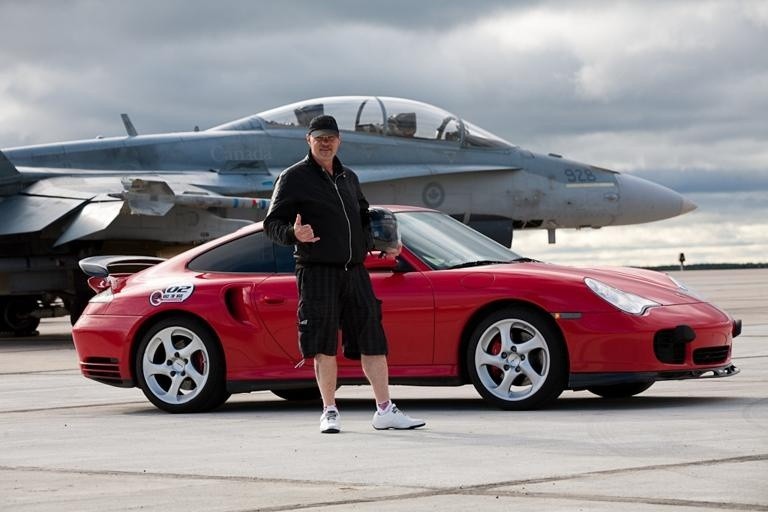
[372,404,426,430]
[320,406,340,433]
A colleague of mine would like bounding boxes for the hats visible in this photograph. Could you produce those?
[308,115,339,137]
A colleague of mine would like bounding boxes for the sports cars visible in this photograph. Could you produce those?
[71,201,745,415]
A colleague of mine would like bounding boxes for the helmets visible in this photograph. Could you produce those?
[368,208,400,252]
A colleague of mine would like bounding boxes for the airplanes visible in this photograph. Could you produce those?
[1,93,704,340]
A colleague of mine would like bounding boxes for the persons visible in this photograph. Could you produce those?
[263,115,425,433]
